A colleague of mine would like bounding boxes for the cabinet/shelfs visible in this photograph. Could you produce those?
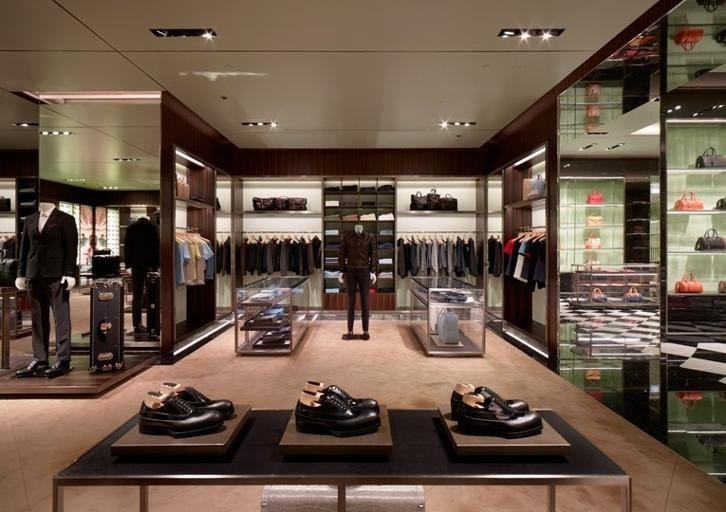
[16,178,38,265]
[559,79,625,141]
[668,22,725,67]
[569,263,658,311]
[410,276,486,357]
[323,177,396,310]
[560,174,623,287]
[667,121,726,329]
[233,274,313,356]
[623,180,650,260]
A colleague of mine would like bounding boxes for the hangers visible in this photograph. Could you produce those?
[174,225,203,237]
[488,232,503,243]
[398,232,481,244]
[216,232,231,244]
[516,225,546,239]
[243,232,323,240]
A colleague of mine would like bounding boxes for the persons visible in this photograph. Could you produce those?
[14,202,78,379]
[338,224,376,340]
[125,218,161,341]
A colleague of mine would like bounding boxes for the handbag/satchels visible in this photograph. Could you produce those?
[586,84,601,96]
[676,390,704,409]
[624,286,643,302]
[630,245,647,259]
[633,225,645,233]
[675,191,705,211]
[410,192,427,210]
[274,198,288,210]
[587,105,601,122]
[0,249,17,286]
[675,29,704,52]
[439,194,458,211]
[253,197,275,210]
[720,280,726,293]
[588,287,607,304]
[695,147,725,167]
[675,273,703,294]
[586,371,601,380]
[437,307,458,344]
[0,196,11,211]
[696,0,725,13]
[287,198,307,210]
[695,228,726,251]
[586,233,601,248]
[586,212,603,227]
[589,188,604,204]
[427,189,440,210]
[717,197,726,210]
[696,435,725,454]
[585,255,600,271]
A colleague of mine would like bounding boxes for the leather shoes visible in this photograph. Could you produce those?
[458,393,543,439]
[362,333,369,340]
[133,324,145,336]
[295,393,381,437]
[303,384,379,414]
[450,387,529,420]
[347,331,353,339]
[146,325,160,335]
[15,360,49,378]
[158,385,233,419]
[43,360,70,377]
[138,393,223,438]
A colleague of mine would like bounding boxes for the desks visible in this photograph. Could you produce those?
[54,410,631,512]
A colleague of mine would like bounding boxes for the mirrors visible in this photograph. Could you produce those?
[558,19,659,143]
[664,0,726,95]
[37,103,160,192]
[216,169,236,320]
[487,167,503,320]
